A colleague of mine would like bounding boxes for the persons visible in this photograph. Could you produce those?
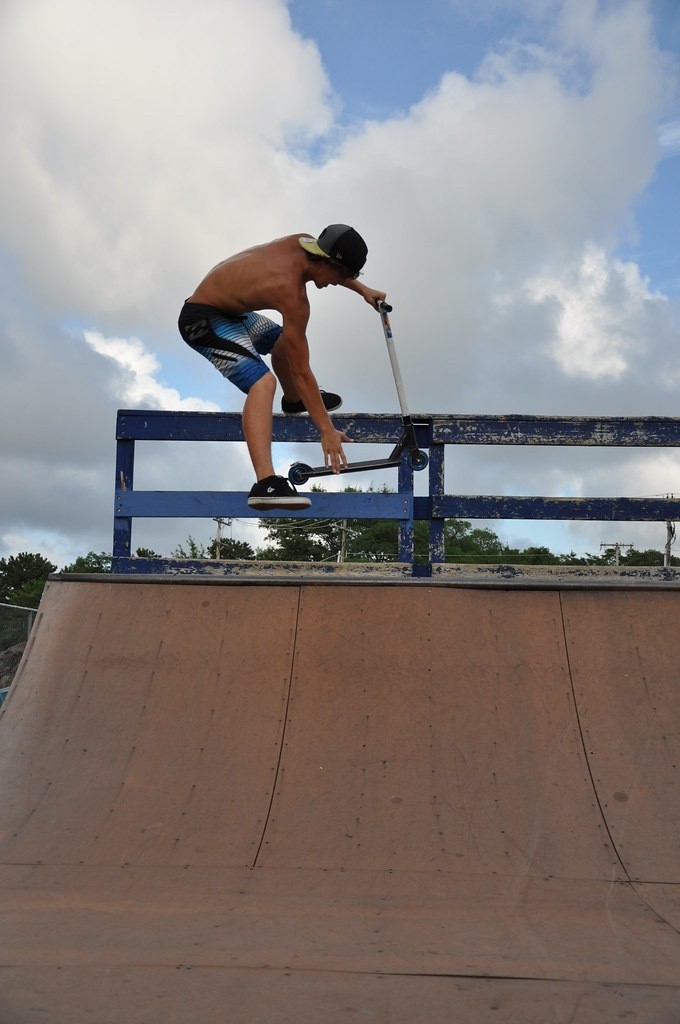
[179,223,387,512]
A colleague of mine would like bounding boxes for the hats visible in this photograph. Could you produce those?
[299,224,368,273]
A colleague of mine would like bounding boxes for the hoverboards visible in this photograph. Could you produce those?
[287,296,429,485]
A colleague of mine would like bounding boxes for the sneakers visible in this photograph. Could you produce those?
[280,388,344,416]
[246,475,313,511]
